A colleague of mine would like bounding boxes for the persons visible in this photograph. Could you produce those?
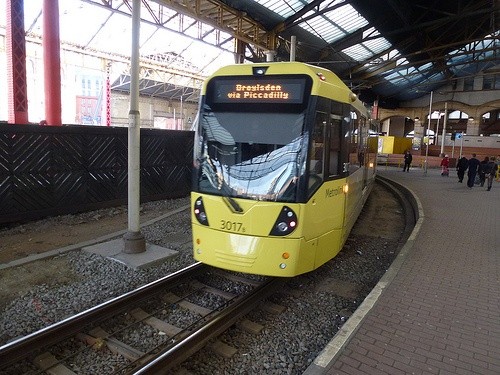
[456,153,469,183]
[402,151,412,173]
[466,153,481,187]
[487,157,498,191]
[478,156,490,187]
[440,155,450,176]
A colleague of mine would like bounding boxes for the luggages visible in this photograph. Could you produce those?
[440,166,449,177]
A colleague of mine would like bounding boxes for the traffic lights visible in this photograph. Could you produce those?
[450,132,455,140]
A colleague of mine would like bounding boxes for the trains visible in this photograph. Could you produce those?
[190,61,379,278]
